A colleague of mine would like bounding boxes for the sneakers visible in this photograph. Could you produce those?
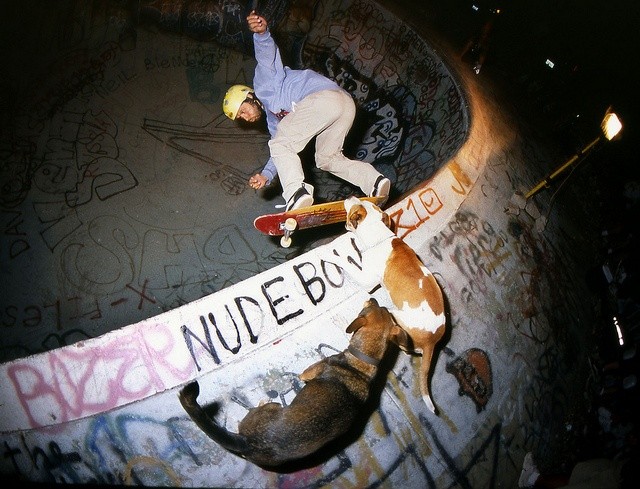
[285,187,314,213]
[368,175,391,198]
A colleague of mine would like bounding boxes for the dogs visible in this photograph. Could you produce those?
[344,195,446,415]
[178,298,410,473]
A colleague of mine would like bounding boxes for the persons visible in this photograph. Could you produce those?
[518,451,640,488]
[222,9,392,211]
[566,222,639,451]
[458,20,493,75]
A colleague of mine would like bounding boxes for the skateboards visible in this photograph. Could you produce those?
[253,196,385,247]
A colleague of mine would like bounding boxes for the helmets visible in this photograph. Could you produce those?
[222,84,255,120]
